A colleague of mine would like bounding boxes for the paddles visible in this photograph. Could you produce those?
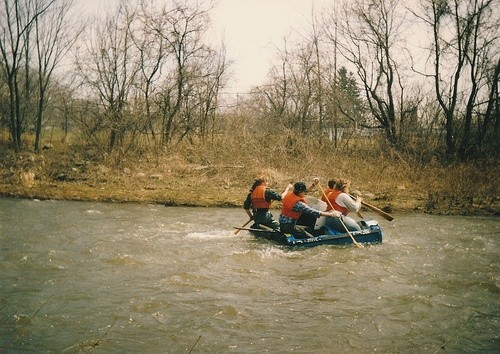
[315,179,364,248]
[235,189,286,235]
[349,195,394,222]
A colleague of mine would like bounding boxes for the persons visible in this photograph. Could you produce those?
[244,176,292,229]
[321,179,337,202]
[325,179,362,231]
[278,182,341,236]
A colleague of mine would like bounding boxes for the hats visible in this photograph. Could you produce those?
[295,182,309,192]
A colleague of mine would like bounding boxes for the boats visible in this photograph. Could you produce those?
[248,219,383,246]
